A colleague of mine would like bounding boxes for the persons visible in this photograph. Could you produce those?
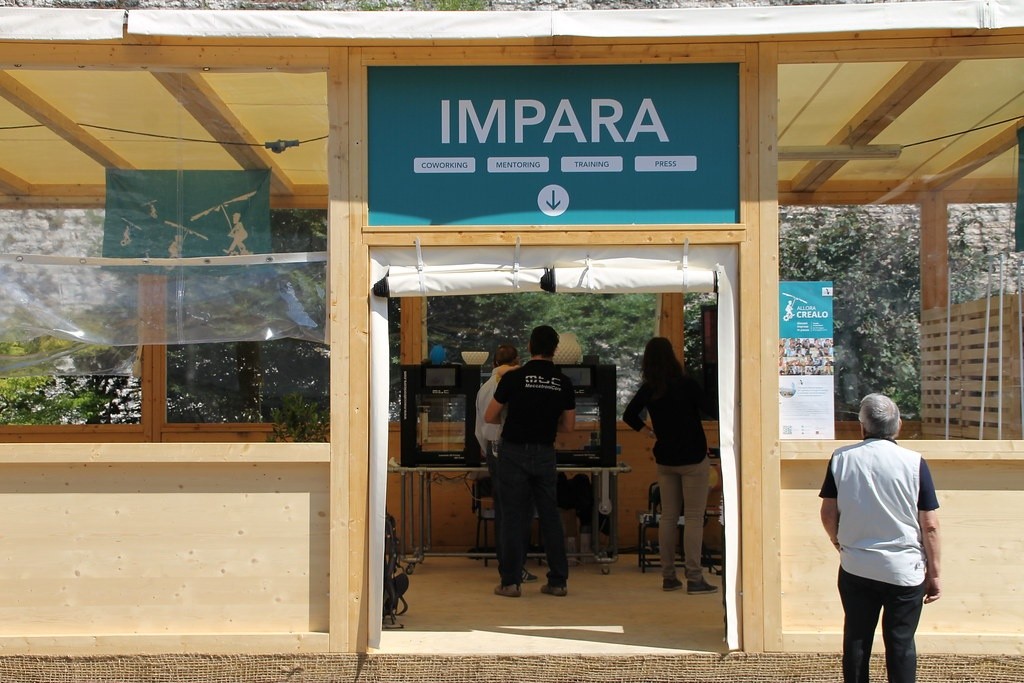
[475,342,538,581]
[484,325,575,598]
[555,468,618,562]
[779,338,833,375]
[623,338,719,590]
[818,392,941,683]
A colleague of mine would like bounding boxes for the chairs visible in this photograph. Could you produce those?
[637,482,708,573]
[472,472,496,568]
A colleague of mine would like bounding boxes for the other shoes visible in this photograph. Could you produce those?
[663,578,683,590]
[687,575,718,595]
[495,584,521,597]
[520,566,538,583]
[541,583,567,596]
